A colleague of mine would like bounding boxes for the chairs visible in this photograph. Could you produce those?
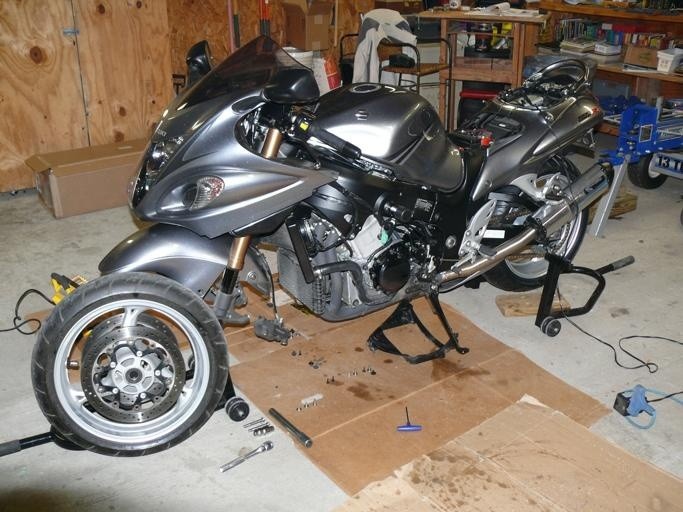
[357,9,451,134]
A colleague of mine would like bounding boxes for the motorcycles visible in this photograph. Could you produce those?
[30,36,635,456]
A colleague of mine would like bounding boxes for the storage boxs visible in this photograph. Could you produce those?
[23,137,149,220]
[278,1,333,52]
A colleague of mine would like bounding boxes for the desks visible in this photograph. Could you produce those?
[416,7,549,142]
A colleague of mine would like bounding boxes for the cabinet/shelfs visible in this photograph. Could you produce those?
[522,0,682,154]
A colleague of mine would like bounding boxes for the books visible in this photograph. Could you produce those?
[559,37,622,61]
[622,46,657,73]
[484,2,539,18]
